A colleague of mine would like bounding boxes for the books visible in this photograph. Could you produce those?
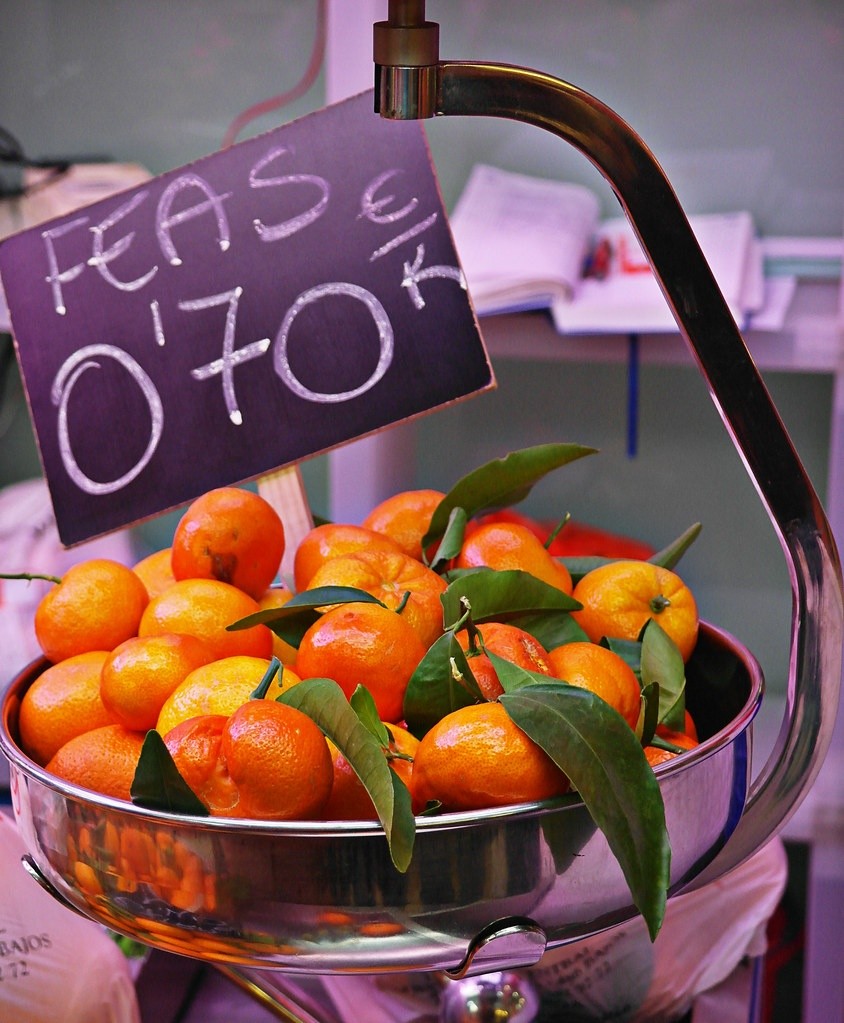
[448,162,765,336]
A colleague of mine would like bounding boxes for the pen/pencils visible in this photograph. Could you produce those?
[591,239,609,280]
[581,236,596,278]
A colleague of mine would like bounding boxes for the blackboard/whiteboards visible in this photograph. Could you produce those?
[0,79,499,551]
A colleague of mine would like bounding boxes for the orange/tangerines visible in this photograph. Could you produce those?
[19,488,699,819]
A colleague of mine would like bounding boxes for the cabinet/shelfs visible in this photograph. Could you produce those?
[0,0,844,1023]
